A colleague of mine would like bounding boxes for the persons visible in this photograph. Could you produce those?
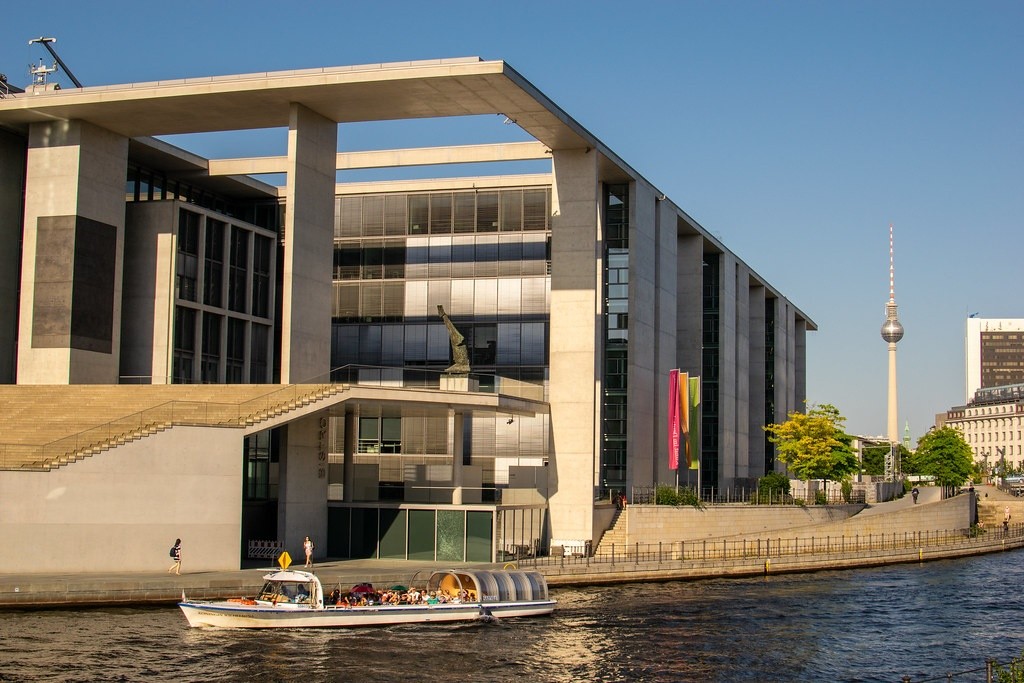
[978,520,983,529]
[259,582,476,606]
[168,538,182,575]
[975,491,980,501]
[616,493,627,510]
[968,484,974,492]
[912,487,919,504]
[304,536,314,568]
[1004,505,1010,522]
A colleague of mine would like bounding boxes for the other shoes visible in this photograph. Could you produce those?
[304,565,307,569]
[310,564,313,568]
[168,569,171,575]
[175,572,181,575]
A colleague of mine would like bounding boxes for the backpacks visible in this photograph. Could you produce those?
[170,547,176,557]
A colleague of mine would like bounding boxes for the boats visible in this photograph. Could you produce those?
[177,547,558,630]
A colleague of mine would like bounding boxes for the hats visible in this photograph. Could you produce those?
[334,589,339,596]
[431,591,436,596]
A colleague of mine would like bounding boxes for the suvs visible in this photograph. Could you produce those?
[1003,473,1024,484]
